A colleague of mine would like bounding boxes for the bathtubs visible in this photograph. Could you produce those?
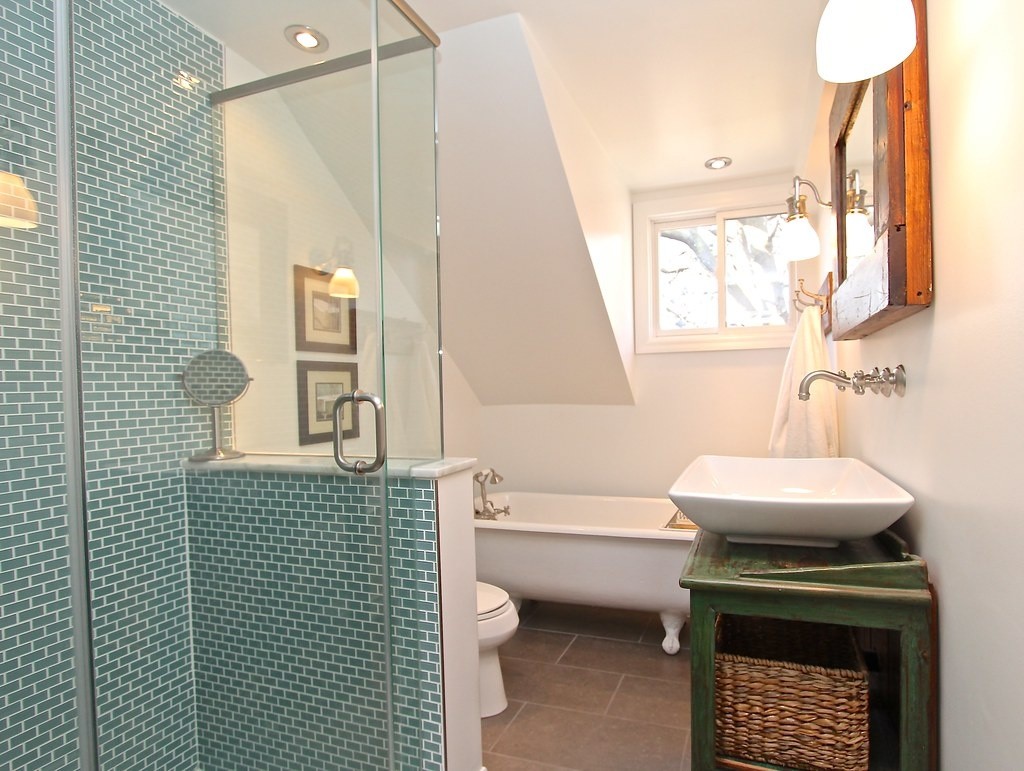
[470,487,700,658]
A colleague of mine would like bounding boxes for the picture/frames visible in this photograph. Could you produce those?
[293,263,357,356]
[296,359,360,446]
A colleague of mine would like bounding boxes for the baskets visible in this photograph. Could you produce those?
[713,612,872,771]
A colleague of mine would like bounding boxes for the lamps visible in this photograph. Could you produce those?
[327,266,360,299]
[817,0,918,85]
[846,169,875,258]
[285,24,331,54]
[775,176,831,263]
[0,170,40,230]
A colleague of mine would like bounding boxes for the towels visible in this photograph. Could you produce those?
[768,305,843,458]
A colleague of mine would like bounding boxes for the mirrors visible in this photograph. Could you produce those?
[828,3,934,344]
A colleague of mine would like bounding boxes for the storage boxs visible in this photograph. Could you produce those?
[712,611,868,770]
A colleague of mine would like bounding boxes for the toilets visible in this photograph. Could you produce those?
[475,575,524,725]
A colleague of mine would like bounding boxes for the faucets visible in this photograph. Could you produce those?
[489,504,511,518]
[794,363,909,405]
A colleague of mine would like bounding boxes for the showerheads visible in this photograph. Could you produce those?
[472,468,504,484]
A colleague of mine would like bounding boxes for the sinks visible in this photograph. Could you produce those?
[667,451,919,556]
